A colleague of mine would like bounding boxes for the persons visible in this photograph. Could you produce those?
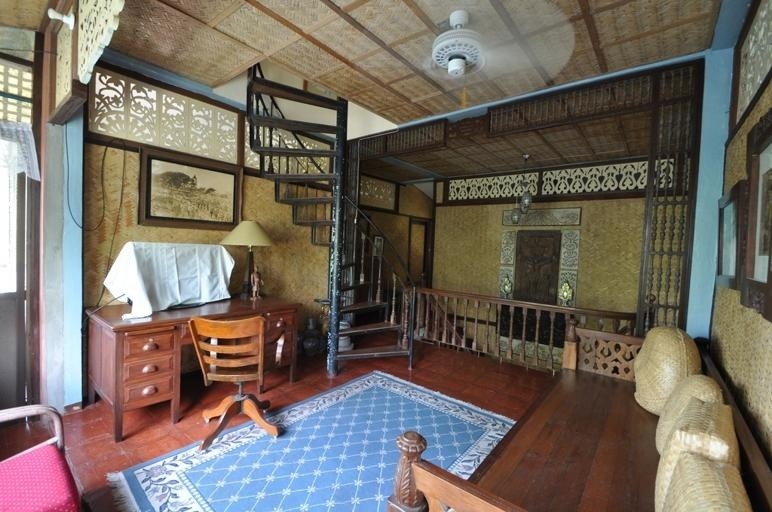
[250,265,265,302]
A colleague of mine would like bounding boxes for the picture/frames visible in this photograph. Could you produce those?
[137,143,245,231]
[740,108,772,321]
[714,181,751,291]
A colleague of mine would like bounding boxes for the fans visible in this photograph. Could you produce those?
[349,0,577,106]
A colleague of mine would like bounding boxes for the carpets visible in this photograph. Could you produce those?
[104,368,519,512]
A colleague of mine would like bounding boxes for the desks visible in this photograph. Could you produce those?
[84,294,304,444]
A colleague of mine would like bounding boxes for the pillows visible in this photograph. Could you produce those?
[661,452,756,511]
[654,375,724,450]
[652,397,742,511]
[631,323,702,414]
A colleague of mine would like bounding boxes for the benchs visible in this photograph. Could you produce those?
[386,319,772,511]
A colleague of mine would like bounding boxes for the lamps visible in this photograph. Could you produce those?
[510,195,520,225]
[519,154,533,214]
[220,220,274,304]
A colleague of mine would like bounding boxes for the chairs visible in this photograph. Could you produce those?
[0,404,86,512]
[187,316,284,453]
